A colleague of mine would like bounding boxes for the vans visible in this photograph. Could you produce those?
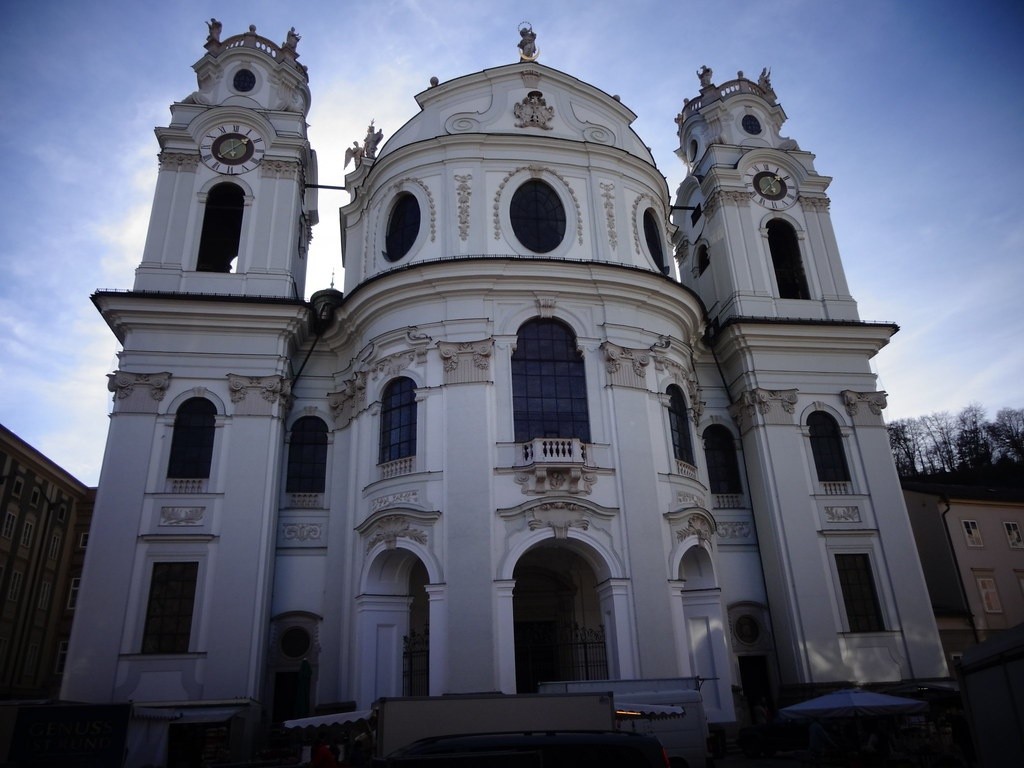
[615,690,713,768]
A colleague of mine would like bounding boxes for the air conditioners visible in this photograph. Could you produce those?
[727,612,772,652]
[274,623,318,664]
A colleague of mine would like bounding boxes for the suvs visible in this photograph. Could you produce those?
[338,730,691,768]
[735,707,834,759]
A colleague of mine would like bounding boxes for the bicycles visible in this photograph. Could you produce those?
[800,742,849,768]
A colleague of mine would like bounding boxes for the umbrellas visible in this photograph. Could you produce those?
[778,687,929,745]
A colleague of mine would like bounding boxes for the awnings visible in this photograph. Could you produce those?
[614,703,687,720]
[281,710,377,735]
[171,708,238,724]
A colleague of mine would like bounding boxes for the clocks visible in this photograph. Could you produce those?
[744,161,799,211]
[199,124,265,175]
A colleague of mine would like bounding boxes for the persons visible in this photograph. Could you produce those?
[518,28,536,62]
[287,27,302,52]
[757,67,771,92]
[344,127,383,167]
[224,746,232,764]
[205,18,222,41]
[809,712,897,768]
[307,728,376,767]
[944,713,978,768]
[697,65,712,87]
